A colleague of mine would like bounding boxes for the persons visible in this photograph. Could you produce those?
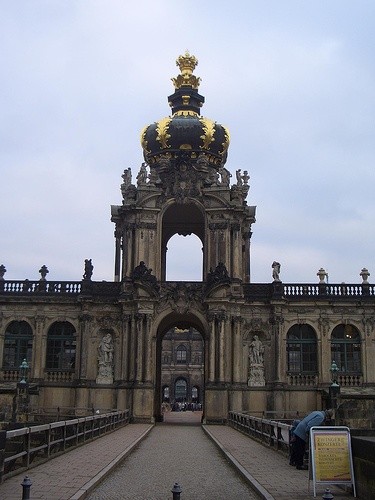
[271,262,282,281]
[235,169,243,188]
[161,399,203,414]
[84,259,93,278]
[250,335,265,364]
[217,168,230,187]
[134,261,157,284]
[99,333,113,364]
[139,163,149,183]
[204,263,228,283]
[290,409,335,470]
[125,166,132,185]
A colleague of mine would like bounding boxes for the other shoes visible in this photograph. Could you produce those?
[296,465,307,470]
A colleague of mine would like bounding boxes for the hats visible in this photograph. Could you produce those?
[326,409,333,417]
